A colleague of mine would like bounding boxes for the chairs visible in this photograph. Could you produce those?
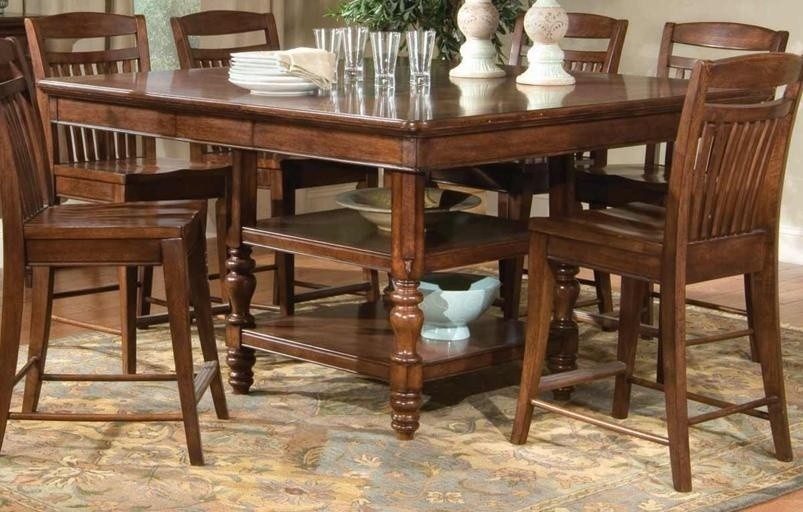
[555,21,791,342]
[22,9,243,375]
[506,51,803,489]
[170,8,380,318]
[4,38,231,466]
[388,10,627,324]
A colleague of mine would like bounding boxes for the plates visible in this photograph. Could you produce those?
[230,47,317,96]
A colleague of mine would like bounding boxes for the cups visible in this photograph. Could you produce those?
[327,76,433,122]
[312,24,435,78]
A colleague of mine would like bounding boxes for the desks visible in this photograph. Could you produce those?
[34,55,766,440]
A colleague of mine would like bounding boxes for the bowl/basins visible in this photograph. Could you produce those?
[403,271,503,340]
[335,186,482,230]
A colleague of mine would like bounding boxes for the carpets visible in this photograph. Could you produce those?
[5,262,803,510]
